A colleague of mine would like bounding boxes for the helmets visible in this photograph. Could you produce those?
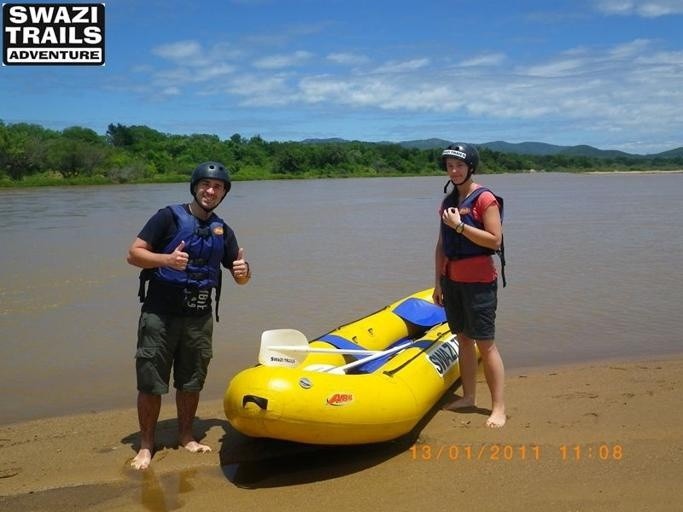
[190,161,231,193]
[442,143,478,172]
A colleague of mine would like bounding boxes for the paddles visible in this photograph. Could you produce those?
[258,329,414,375]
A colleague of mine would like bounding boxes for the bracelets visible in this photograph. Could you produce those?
[244,261,249,278]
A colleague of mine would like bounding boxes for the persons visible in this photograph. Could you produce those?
[432,142,507,429]
[126,162,252,471]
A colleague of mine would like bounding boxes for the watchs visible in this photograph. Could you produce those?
[456,222,466,233]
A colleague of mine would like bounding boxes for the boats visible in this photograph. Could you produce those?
[223,287,481,446]
